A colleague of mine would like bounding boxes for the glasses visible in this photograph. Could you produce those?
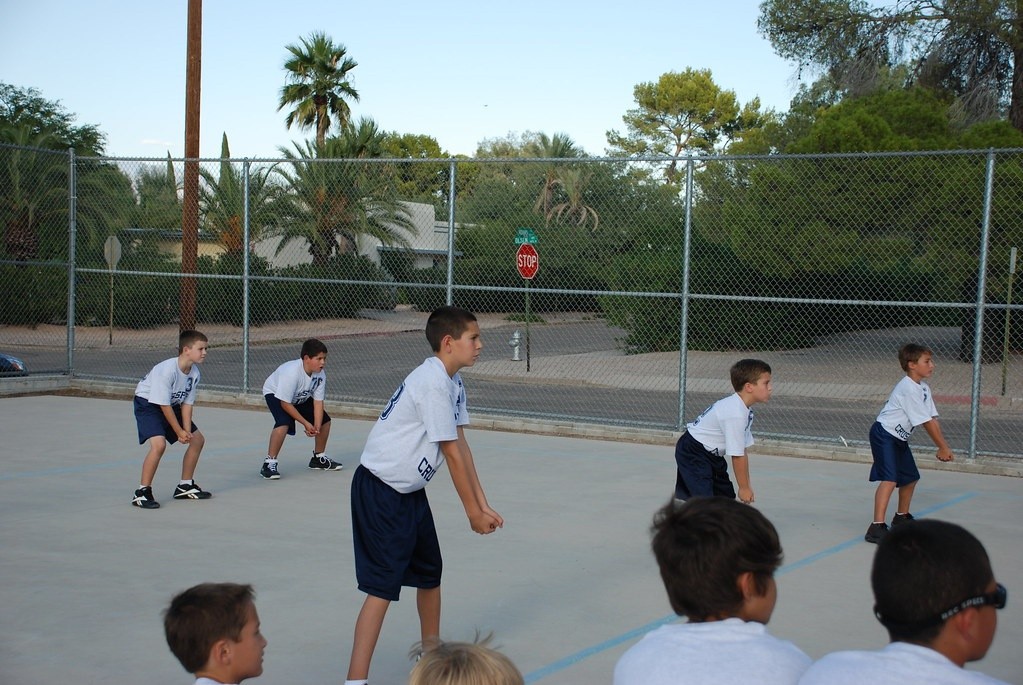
[874,581,1007,632]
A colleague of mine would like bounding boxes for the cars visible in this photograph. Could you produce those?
[0,352,29,378]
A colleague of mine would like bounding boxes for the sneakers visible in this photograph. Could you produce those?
[173,479,212,499]
[891,512,916,527]
[260,455,280,480]
[132,486,160,509]
[308,451,343,471]
[865,522,890,544]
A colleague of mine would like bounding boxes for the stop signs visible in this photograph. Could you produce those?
[515,243,539,279]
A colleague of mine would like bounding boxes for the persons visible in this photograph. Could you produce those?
[865,344,953,543]
[345,306,503,685]
[260,338,343,480]
[410,631,524,685]
[800,519,1008,685]
[674,359,772,503]
[165,583,267,685]
[132,330,212,508]
[614,497,812,685]
